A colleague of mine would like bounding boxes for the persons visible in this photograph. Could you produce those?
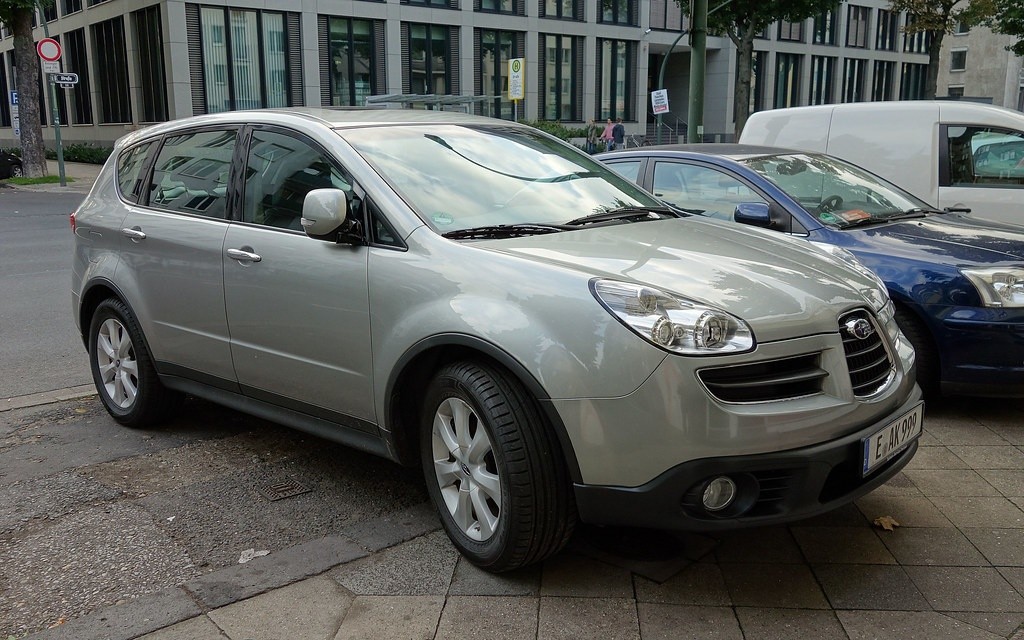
[587,119,596,155]
[599,117,625,151]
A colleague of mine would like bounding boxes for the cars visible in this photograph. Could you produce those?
[522,142,1024,405]
[0,148,23,179]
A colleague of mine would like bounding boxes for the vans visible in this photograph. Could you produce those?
[738,99,1024,236]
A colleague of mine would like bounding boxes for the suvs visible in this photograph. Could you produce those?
[73,104,925,573]
